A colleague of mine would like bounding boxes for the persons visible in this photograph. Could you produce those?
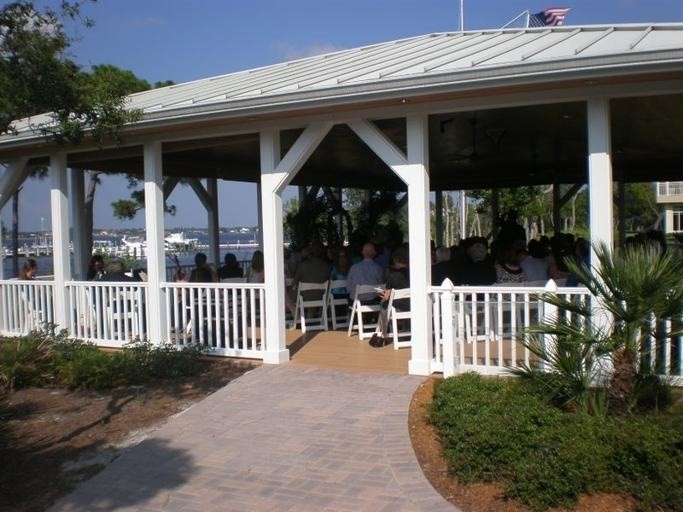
[17,259,38,280]
[285,243,409,340]
[87,255,143,281]
[188,251,263,282]
[431,234,589,286]
[499,209,525,249]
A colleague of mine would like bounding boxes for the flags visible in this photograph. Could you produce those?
[529,8,575,27]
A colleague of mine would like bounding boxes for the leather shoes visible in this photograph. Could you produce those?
[369,333,387,347]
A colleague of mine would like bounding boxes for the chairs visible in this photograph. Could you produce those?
[293,282,330,333]
[382,289,411,348]
[348,284,388,341]
[20,268,296,338]
[428,269,589,344]
[327,278,353,332]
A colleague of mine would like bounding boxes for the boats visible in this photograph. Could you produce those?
[1,229,208,259]
